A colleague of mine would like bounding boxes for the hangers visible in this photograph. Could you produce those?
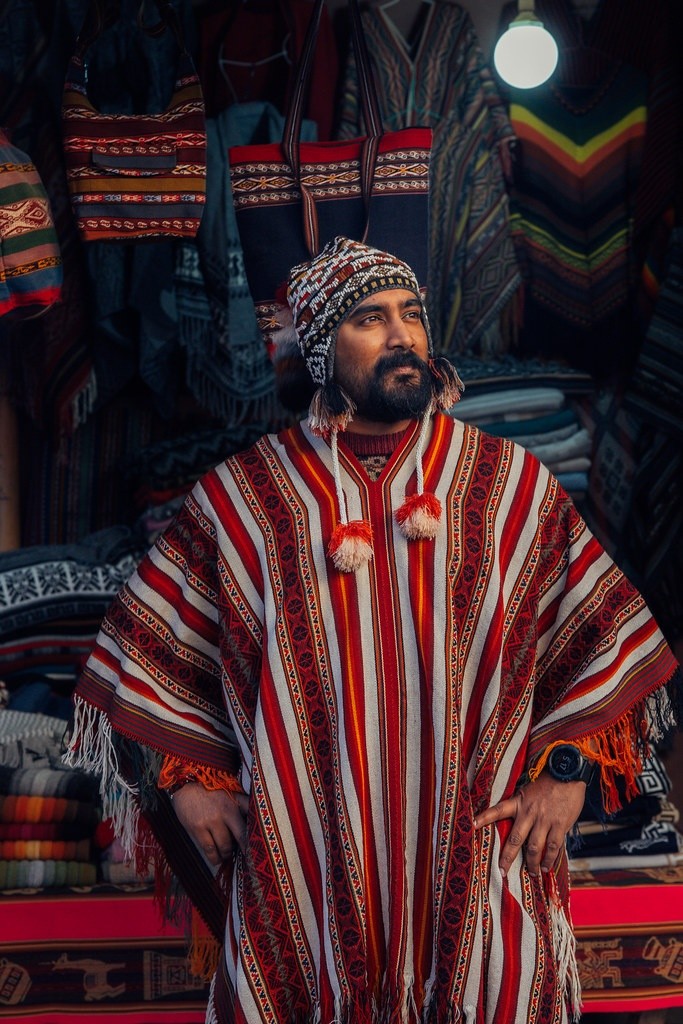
[385,79,443,127]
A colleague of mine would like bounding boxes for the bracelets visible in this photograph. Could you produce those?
[166,774,195,798]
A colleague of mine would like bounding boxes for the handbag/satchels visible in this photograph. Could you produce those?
[0,127,63,316]
[62,0,208,245]
[229,127,435,331]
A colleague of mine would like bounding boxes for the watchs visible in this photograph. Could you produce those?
[550,747,596,786]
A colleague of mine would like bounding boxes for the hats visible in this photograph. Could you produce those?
[287,236,465,437]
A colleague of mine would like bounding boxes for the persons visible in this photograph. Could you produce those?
[61,237,680,1024]
